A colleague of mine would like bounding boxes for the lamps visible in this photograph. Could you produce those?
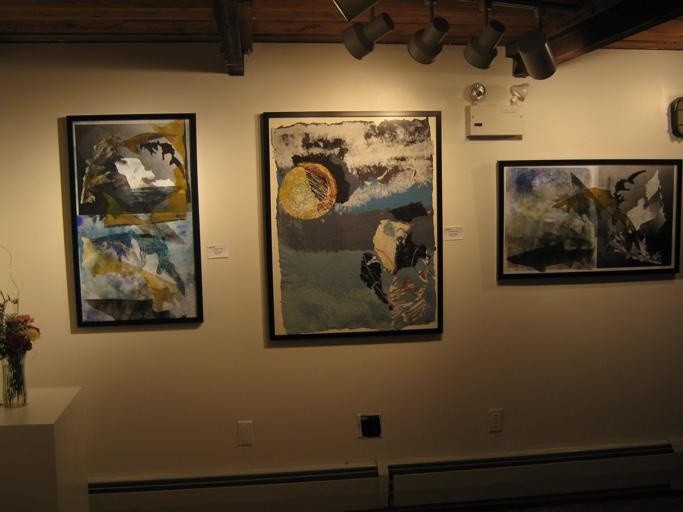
[331,0,557,104]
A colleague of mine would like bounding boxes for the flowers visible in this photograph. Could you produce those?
[0,294,39,407]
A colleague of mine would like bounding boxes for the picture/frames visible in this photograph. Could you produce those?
[260,111,445,342]
[492,158,683,284]
[65,114,204,328]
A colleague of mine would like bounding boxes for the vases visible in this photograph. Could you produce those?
[0,354,27,408]
